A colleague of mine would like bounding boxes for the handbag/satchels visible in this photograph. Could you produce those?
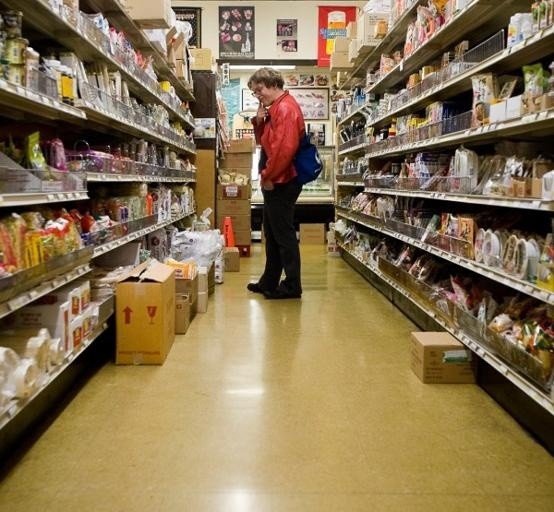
[293,135,322,183]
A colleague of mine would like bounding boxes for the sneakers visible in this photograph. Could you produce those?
[264,285,302,299]
[248,284,279,293]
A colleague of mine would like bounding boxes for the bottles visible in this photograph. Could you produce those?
[399,162,406,179]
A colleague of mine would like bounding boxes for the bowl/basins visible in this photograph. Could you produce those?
[474,228,544,278]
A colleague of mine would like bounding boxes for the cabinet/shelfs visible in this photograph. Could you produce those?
[0,0,196,452]
[331,1,554,460]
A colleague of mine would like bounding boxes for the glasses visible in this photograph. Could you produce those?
[252,92,256,97]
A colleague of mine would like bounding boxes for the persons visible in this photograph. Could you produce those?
[244,68,304,301]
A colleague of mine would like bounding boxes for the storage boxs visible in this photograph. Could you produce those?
[217,138,256,256]
[299,224,326,247]
[187,49,214,72]
[116,258,223,365]
[224,246,241,272]
